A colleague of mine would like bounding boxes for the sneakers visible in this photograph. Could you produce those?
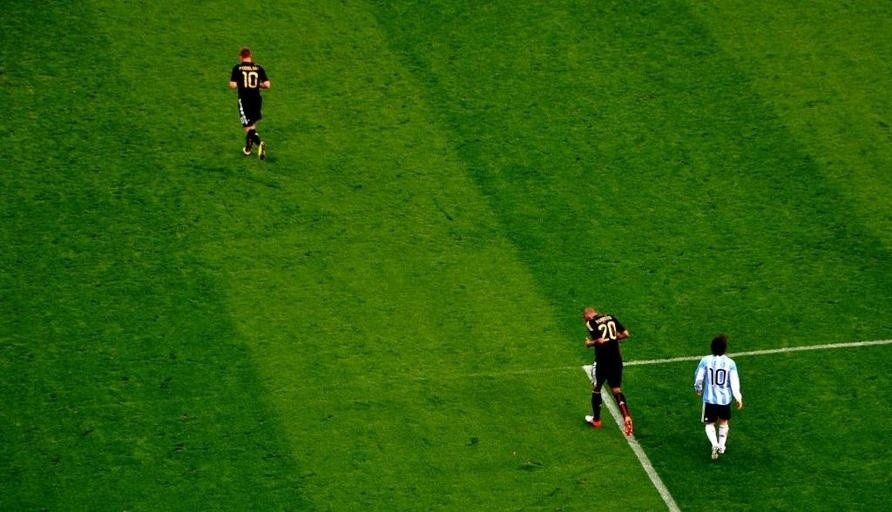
[711,445,719,459]
[258,141,266,160]
[719,447,726,454]
[585,415,601,427]
[624,417,632,436]
[242,147,251,156]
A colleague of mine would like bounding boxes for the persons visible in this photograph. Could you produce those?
[229,48,271,161]
[695,336,743,461]
[582,306,634,437]
